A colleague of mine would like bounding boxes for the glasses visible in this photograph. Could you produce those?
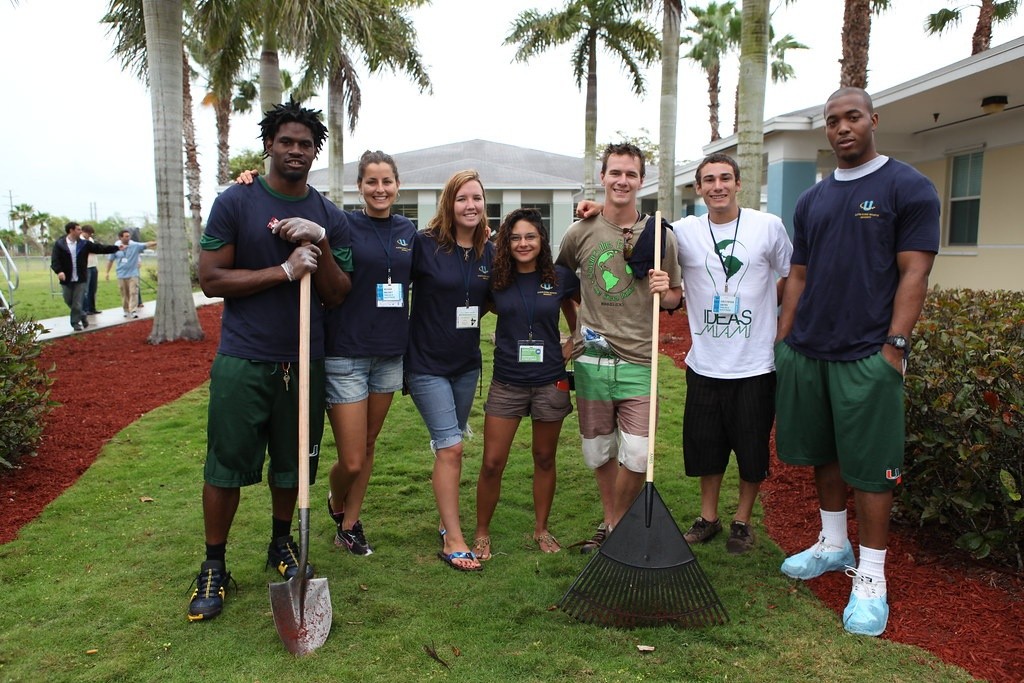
[509,233,542,242]
[622,227,635,262]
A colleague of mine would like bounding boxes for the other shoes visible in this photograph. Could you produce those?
[81,317,89,328]
[73,323,82,331]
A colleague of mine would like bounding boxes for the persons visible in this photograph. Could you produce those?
[576,151,794,551]
[105,230,157,317]
[79,226,102,315]
[469,207,580,561]
[186,100,353,620]
[774,87,942,636]
[401,169,499,571]
[50,222,128,331]
[236,150,418,554]
[554,141,683,555]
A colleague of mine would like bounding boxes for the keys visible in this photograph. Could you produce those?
[283,375,291,391]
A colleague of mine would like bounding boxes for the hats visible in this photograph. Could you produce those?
[81,225,95,234]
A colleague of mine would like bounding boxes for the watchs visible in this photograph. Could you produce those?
[886,334,909,358]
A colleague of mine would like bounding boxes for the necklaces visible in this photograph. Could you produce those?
[458,241,472,262]
[637,213,642,222]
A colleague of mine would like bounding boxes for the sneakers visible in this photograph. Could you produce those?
[332,520,375,558]
[682,515,722,545]
[326,489,345,525]
[579,521,610,556]
[187,560,231,624]
[267,535,315,581]
[780,531,856,580]
[123,311,129,317]
[130,311,139,318]
[842,566,889,637]
[725,518,755,552]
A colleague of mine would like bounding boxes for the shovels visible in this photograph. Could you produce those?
[268,267,333,651]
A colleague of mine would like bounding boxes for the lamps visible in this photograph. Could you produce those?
[981,96,1008,113]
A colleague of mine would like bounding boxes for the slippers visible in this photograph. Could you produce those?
[471,535,492,562]
[437,524,448,548]
[437,550,483,572]
[532,530,562,555]
